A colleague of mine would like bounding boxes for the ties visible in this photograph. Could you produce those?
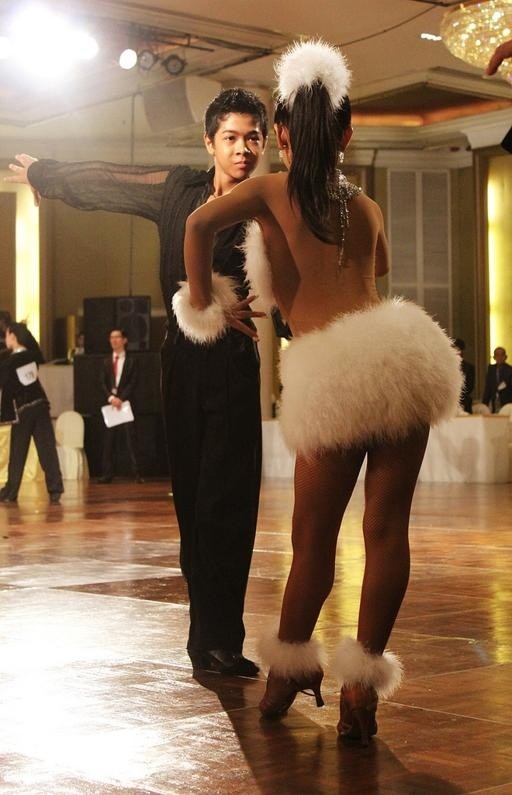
[110,355,120,381]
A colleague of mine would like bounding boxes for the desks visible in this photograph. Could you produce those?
[0,421,45,487]
[259,413,512,484]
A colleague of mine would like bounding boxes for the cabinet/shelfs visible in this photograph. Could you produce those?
[36,356,90,419]
[73,352,169,479]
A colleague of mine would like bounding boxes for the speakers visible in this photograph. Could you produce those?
[84,296,151,353]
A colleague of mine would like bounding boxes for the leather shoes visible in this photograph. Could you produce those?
[98,474,112,483]
[134,474,145,483]
[187,647,257,677]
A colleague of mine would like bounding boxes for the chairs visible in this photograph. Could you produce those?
[471,403,492,414]
[53,411,89,480]
[497,403,512,417]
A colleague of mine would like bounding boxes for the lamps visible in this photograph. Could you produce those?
[116,36,140,76]
[161,54,190,77]
[438,0,512,88]
[139,40,161,71]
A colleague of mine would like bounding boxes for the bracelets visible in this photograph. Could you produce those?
[171,267,242,347]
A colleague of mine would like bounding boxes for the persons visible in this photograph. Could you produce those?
[452,336,475,414]
[170,32,467,749]
[1,322,65,503]
[96,326,147,485]
[0,310,14,359]
[3,87,268,678]
[67,332,84,361]
[481,347,512,413]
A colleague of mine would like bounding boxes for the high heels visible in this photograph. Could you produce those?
[337,680,380,747]
[261,659,324,717]
